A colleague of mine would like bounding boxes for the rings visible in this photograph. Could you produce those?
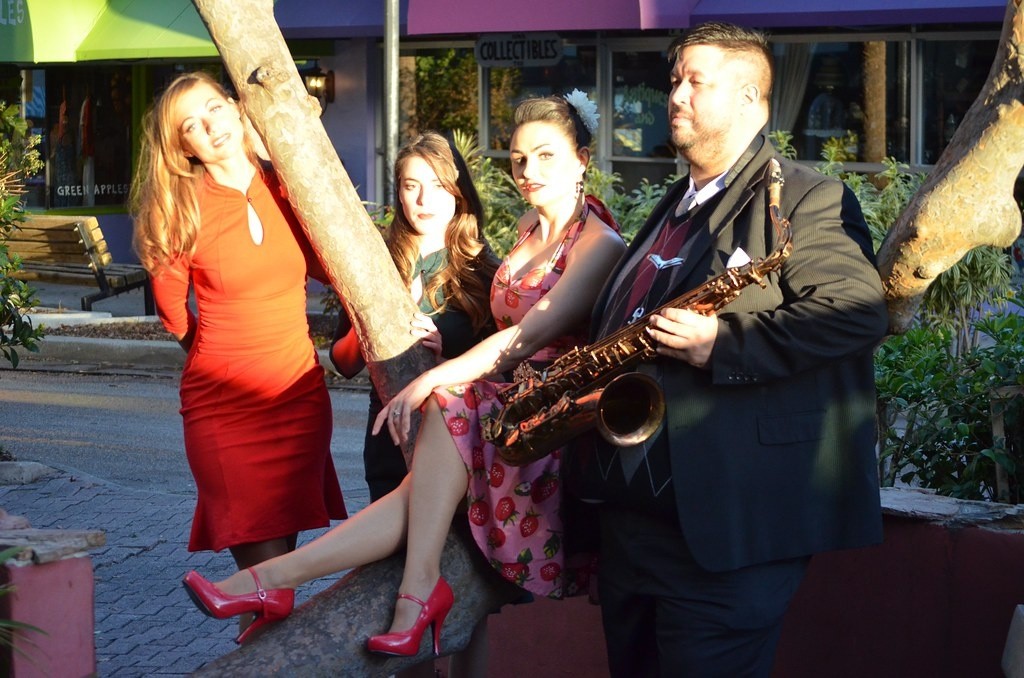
[392,410,401,417]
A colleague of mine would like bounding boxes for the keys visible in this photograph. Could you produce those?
[628,307,644,325]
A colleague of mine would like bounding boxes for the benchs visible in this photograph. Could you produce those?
[1,214,156,315]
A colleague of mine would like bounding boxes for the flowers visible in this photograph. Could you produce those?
[563,87,602,136]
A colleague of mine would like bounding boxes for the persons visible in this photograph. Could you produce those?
[182,95,628,657]
[128,71,349,634]
[592,23,888,678]
[329,130,502,506]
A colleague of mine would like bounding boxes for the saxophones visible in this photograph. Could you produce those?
[483,159,794,468]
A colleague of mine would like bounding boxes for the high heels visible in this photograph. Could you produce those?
[367,575,455,658]
[181,565,297,645]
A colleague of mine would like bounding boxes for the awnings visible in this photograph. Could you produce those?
[0,0,1013,66]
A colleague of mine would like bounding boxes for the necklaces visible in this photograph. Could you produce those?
[419,250,439,274]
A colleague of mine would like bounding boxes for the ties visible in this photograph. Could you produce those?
[675,193,698,218]
